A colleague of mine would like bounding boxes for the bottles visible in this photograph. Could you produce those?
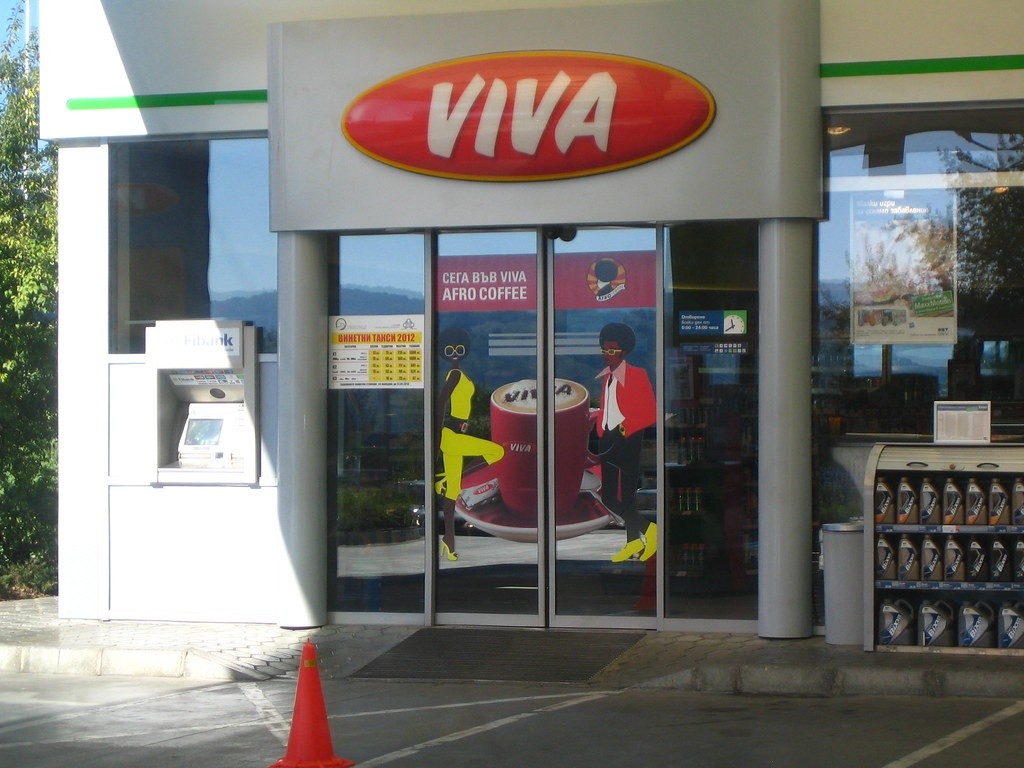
[878,598,916,645]
[957,601,996,648]
[875,532,1024,582]
[917,600,954,646]
[997,601,1024,649]
[875,476,1024,525]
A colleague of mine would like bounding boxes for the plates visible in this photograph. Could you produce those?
[453,465,615,544]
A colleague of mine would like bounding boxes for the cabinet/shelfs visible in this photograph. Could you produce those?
[334,323,1024,660]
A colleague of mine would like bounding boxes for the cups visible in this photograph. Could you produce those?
[489,377,617,521]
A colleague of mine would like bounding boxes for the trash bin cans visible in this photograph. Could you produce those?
[822,522,865,647]
